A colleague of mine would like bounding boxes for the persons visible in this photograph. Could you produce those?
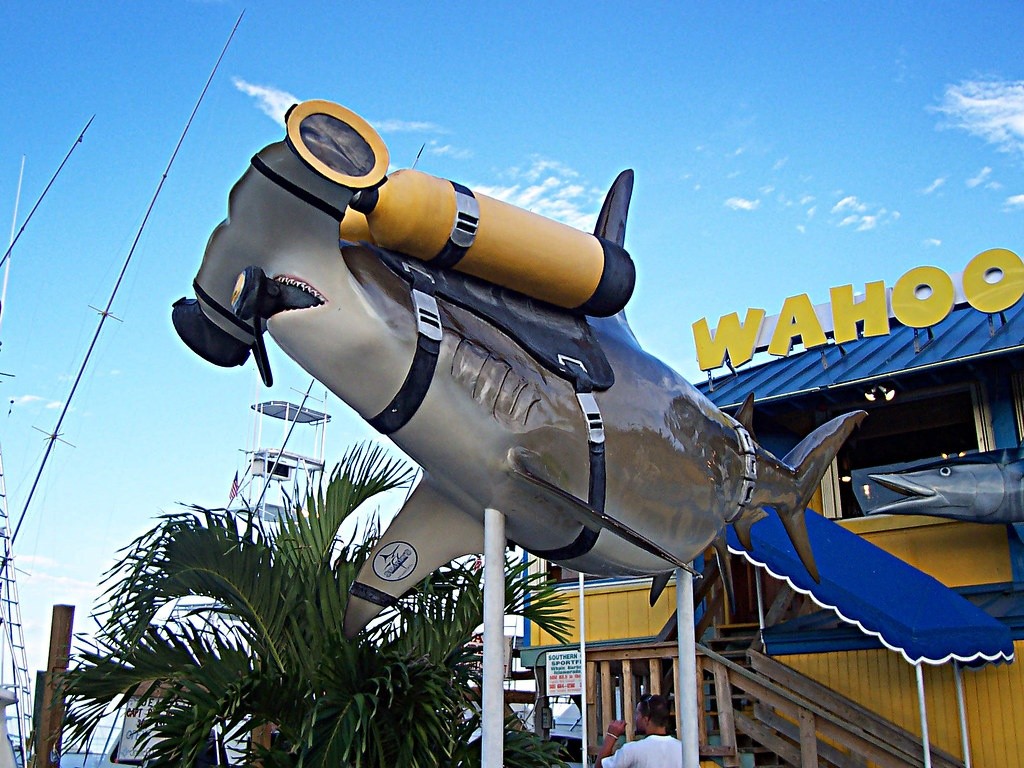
[594,693,701,768]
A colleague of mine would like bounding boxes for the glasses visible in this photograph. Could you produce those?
[644,695,653,714]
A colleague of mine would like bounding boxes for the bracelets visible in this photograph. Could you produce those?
[606,732,618,740]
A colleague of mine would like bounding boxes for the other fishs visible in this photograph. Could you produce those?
[867,437,1024,543]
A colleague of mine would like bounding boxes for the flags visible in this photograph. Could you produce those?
[229,470,238,498]
